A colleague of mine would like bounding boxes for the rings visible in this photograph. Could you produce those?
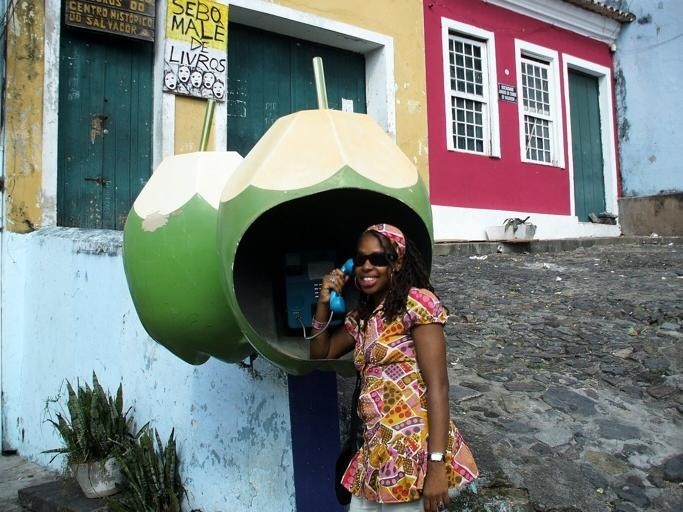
[330,269,335,275]
[329,277,336,282]
[436,501,445,510]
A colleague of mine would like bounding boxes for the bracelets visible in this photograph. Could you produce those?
[312,316,328,332]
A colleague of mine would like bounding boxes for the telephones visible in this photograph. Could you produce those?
[331,259,357,313]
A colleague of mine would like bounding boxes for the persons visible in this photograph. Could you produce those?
[310,224,478,512]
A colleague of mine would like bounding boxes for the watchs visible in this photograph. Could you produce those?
[425,452,447,462]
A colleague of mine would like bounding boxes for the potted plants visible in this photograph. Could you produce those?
[487,215,536,240]
[38,373,149,498]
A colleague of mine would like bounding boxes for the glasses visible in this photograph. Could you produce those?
[355,251,390,267]
